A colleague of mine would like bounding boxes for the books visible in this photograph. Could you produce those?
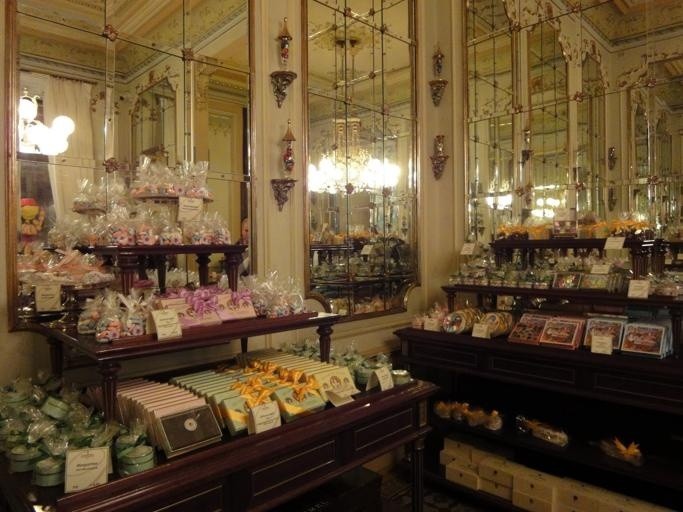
[79,348,363,460]
[506,312,675,360]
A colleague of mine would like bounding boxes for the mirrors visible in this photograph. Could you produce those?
[466,0,520,241]
[627,83,651,220]
[656,112,672,224]
[580,38,607,220]
[527,2,571,221]
[301,1,422,324]
[5,0,257,335]
[131,75,177,170]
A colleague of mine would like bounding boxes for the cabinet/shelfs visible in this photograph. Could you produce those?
[0,243,439,512]
[309,238,418,317]
[392,236,683,511]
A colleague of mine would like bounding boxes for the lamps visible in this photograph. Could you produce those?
[530,19,567,219]
[19,88,75,157]
[270,118,300,211]
[309,25,399,200]
[483,2,513,212]
[428,42,449,106]
[517,181,533,205]
[269,18,296,108]
[522,123,531,169]
[608,181,618,212]
[428,134,449,180]
[607,143,617,171]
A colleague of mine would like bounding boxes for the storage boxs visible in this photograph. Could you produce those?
[440,435,677,511]
